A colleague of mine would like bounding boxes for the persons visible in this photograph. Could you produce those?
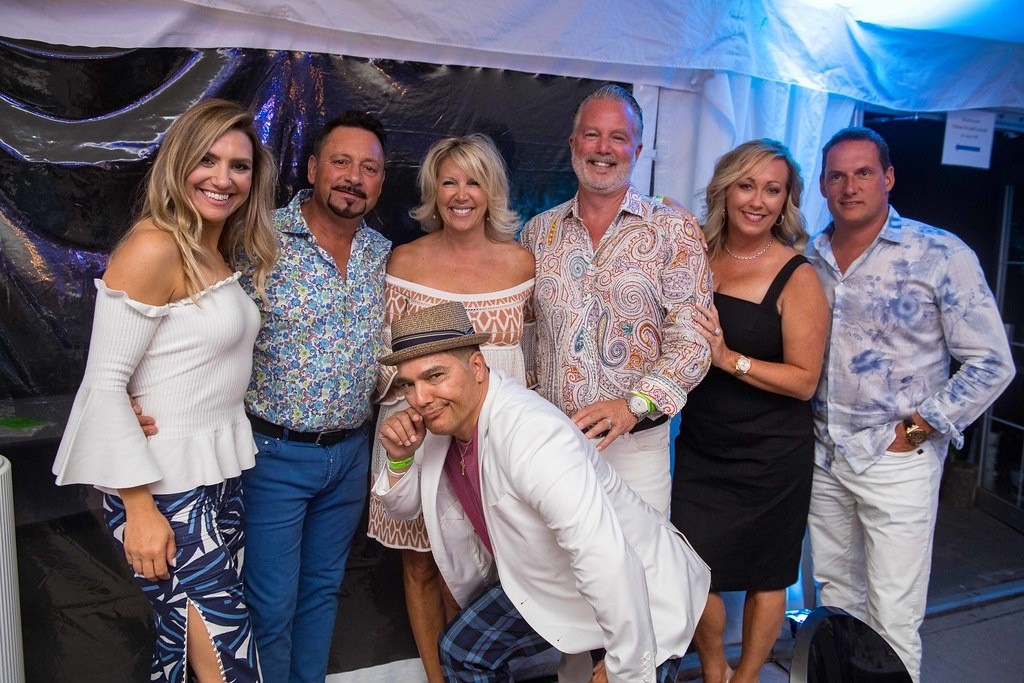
[372,301,712,683]
[670,140,832,683]
[518,84,714,683]
[127,109,393,683]
[365,134,536,683]
[52,98,263,683]
[803,126,1016,683]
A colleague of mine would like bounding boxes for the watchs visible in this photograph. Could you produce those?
[903,416,927,447]
[622,394,648,423]
[732,355,752,378]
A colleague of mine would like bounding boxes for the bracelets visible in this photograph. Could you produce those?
[387,454,415,478]
[655,195,665,203]
[628,389,655,414]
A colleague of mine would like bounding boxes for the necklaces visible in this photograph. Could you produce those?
[724,235,774,260]
[455,437,472,476]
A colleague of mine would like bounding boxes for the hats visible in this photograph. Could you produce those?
[378,302,493,366]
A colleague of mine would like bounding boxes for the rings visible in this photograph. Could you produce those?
[692,217,697,220]
[604,418,613,429]
[712,327,721,336]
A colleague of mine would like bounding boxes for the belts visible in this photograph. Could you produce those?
[580,412,670,438]
[245,412,366,447]
